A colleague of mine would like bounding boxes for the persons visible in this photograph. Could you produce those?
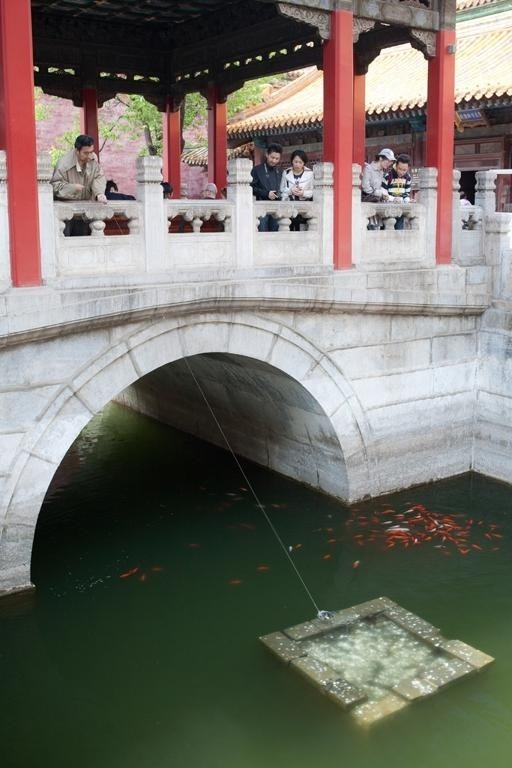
[179,182,218,232]
[50,135,108,234]
[460,192,471,206]
[280,149,314,230]
[249,144,284,231]
[361,148,402,230]
[104,180,135,199]
[163,182,174,199]
[386,154,414,229]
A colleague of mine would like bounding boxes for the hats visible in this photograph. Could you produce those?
[377,147,398,164]
